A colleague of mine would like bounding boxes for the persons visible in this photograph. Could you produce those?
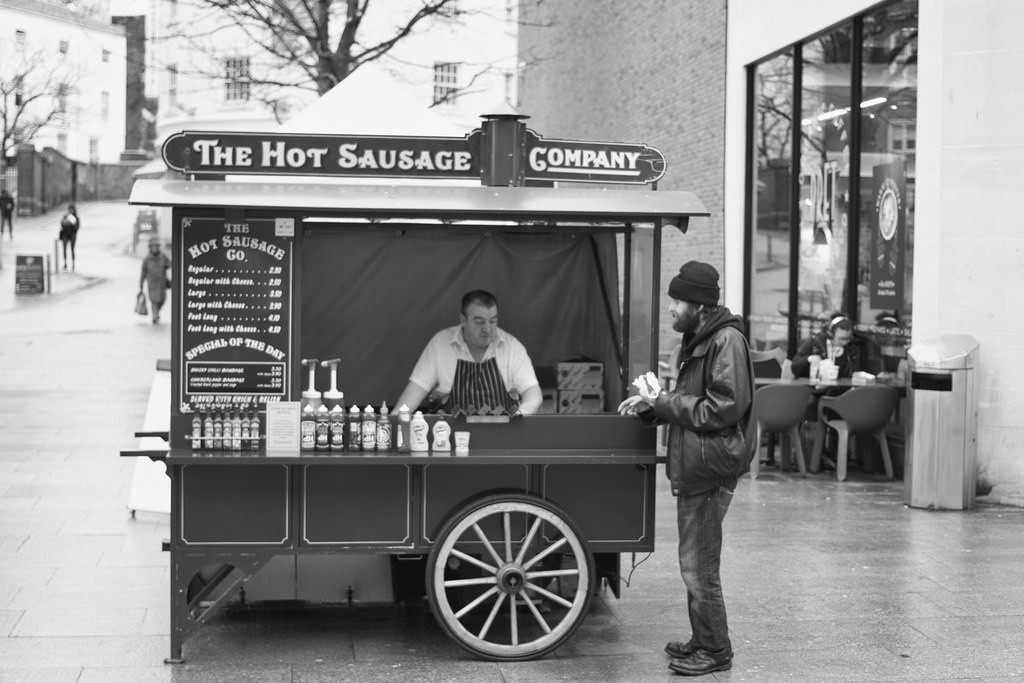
[617,260,758,675]
[61,205,79,270]
[140,237,172,323]
[790,313,867,466]
[389,289,543,415]
[0,188,15,238]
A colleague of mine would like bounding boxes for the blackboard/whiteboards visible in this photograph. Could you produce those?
[14,256,45,296]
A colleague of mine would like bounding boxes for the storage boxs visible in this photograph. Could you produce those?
[549,350,604,391]
[533,367,558,415]
[560,390,605,415]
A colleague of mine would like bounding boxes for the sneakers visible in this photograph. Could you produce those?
[668,648,734,676]
[664,640,698,659]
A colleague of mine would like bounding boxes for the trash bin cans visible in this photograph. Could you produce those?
[903,333,981,510]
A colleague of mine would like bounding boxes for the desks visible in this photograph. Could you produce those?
[755,377,908,472]
[783,287,870,341]
[778,310,827,348]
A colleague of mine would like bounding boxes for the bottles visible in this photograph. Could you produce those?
[204,407,213,450]
[241,406,251,451]
[330,405,345,451]
[377,401,392,451]
[348,405,362,451]
[432,417,451,452]
[301,405,316,451]
[191,406,202,450]
[214,407,223,451]
[316,405,330,452]
[362,405,377,452]
[251,406,260,450]
[223,407,232,451]
[397,405,410,453]
[410,411,429,451]
[232,407,241,451]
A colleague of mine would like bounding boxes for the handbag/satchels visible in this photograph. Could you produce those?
[60,231,63,239]
[135,293,147,314]
[166,279,170,288]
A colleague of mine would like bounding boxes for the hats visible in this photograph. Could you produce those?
[148,237,160,247]
[668,260,721,307]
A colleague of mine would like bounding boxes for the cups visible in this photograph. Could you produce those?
[828,366,839,380]
[454,432,470,452]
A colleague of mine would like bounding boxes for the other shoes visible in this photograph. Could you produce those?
[821,449,838,471]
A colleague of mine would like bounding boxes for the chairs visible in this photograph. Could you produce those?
[806,384,900,481]
[752,357,783,465]
[749,384,814,481]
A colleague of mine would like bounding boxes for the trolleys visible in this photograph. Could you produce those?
[117,114,711,668]
